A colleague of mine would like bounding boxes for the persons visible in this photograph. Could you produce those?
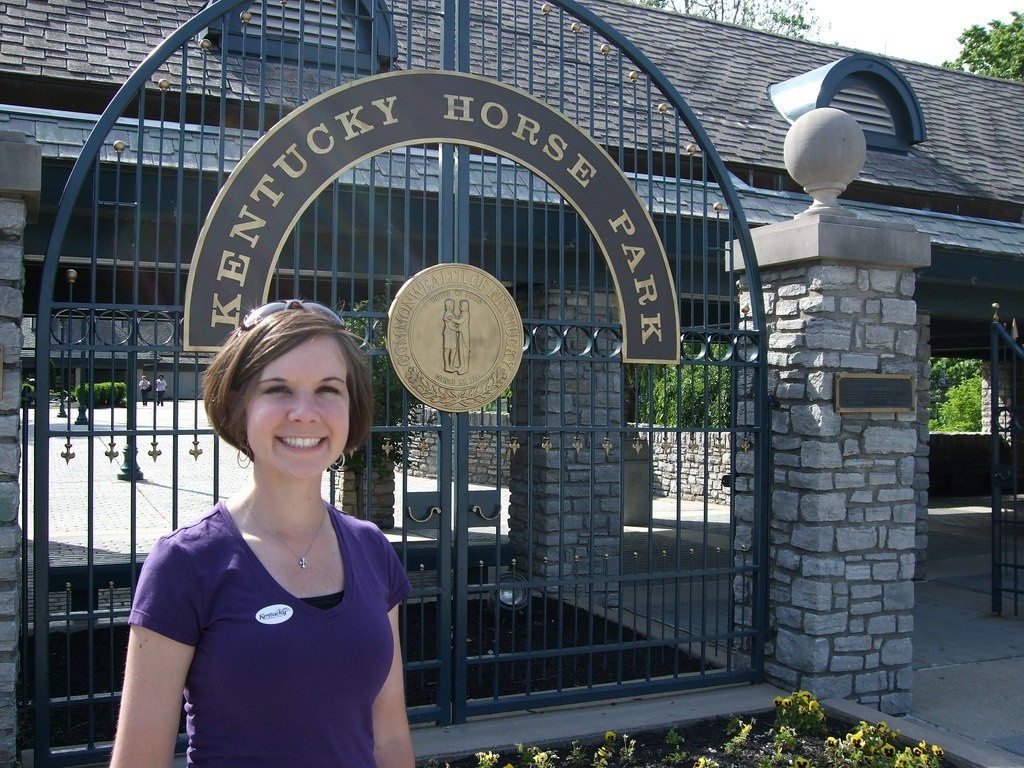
[442,299,470,374]
[139,375,150,406]
[156,374,167,406]
[110,299,418,768]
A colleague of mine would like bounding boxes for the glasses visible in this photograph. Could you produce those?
[237,299,347,340]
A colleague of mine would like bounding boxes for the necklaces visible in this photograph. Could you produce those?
[240,491,325,569]
